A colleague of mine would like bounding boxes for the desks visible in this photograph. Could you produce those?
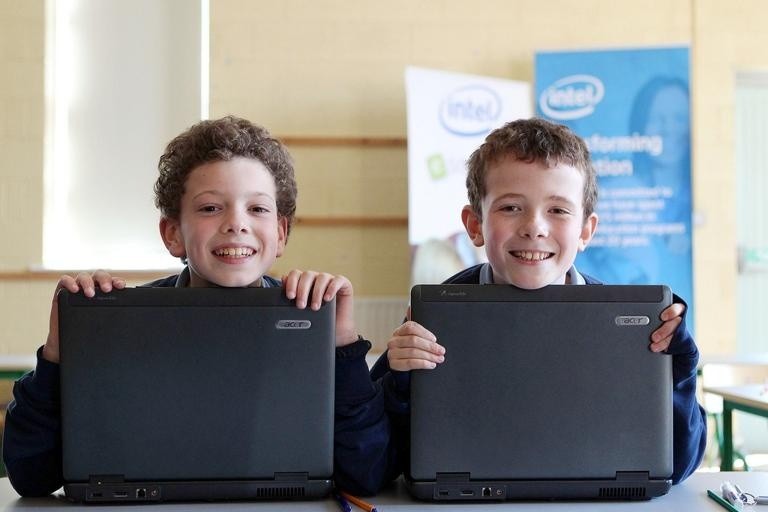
[701,383,768,469]
[0,470,768,512]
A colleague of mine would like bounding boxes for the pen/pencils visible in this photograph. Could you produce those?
[707,490,741,512]
[333,490,378,512]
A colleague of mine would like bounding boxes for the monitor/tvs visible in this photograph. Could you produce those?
[409,282,675,503]
[57,284,338,505]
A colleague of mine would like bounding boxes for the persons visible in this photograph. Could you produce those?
[1,114,396,497]
[368,117,710,486]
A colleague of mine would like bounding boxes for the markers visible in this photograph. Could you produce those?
[721,482,743,509]
